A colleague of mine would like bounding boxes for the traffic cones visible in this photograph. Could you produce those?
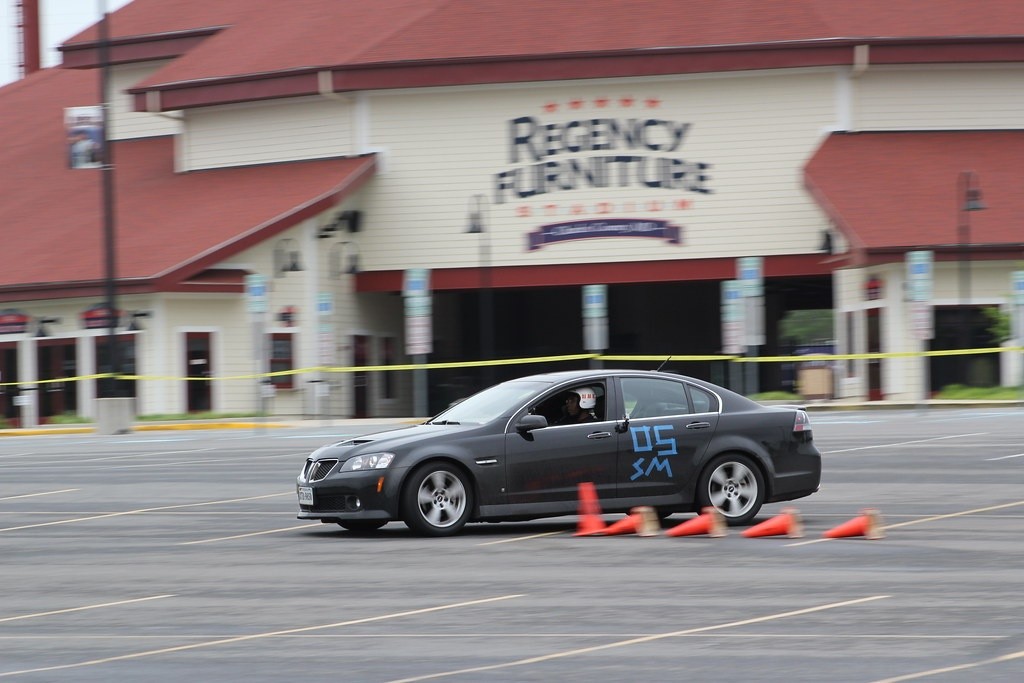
[588,503,659,537]
[661,505,727,538]
[738,507,805,539]
[568,481,607,536]
[822,509,880,540]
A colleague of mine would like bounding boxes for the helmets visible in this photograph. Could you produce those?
[569,386,596,409]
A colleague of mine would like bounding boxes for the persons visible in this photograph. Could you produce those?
[550,392,594,425]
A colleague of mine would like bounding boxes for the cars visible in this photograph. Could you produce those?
[297,368,825,537]
